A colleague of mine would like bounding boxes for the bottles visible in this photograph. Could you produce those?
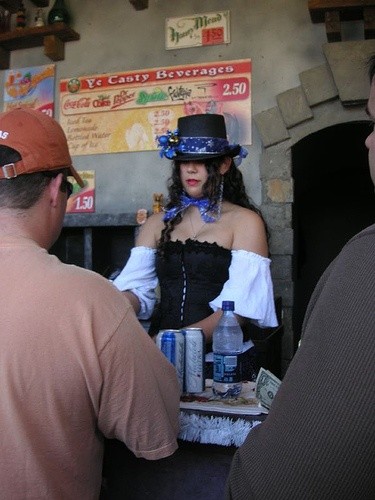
[212,300,243,398]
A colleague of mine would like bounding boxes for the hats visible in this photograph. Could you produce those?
[157,113,242,161]
[0,106,86,189]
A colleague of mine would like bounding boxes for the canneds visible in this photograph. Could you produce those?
[155,329,184,397]
[181,328,205,394]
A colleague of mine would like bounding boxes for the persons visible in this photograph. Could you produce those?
[0,107,181,500]
[225,49,374,500]
[110,114,278,385]
